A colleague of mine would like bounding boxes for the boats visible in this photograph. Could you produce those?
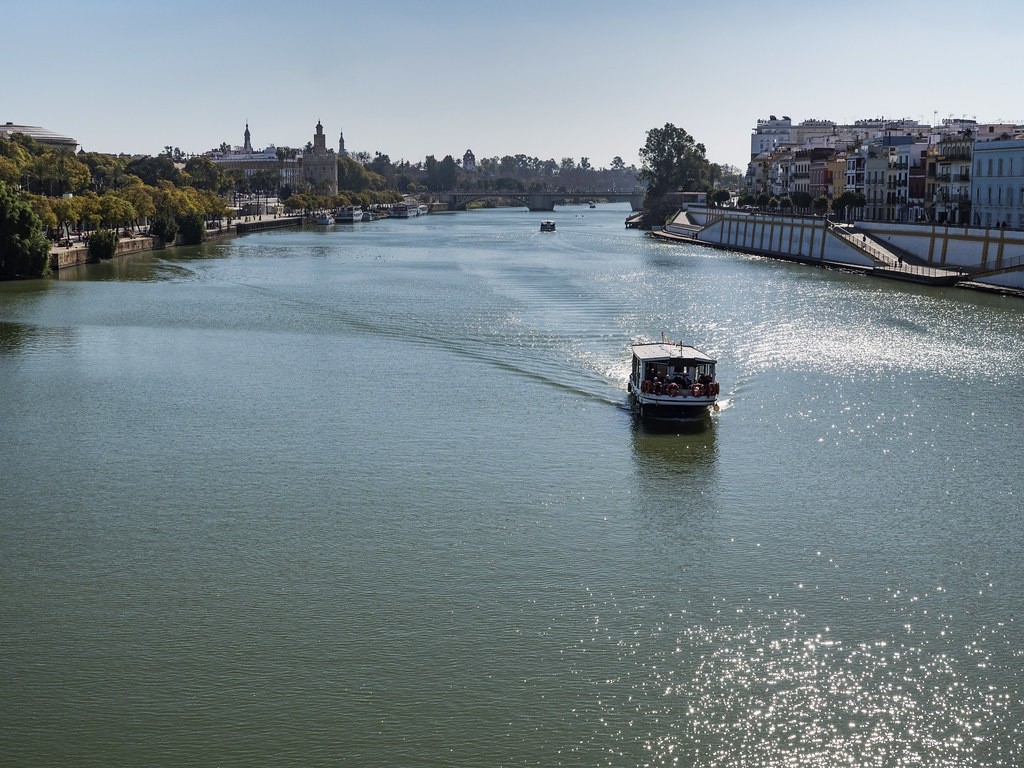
[628,340,717,433]
[540,220,556,232]
[392,201,417,218]
[317,213,335,226]
[336,205,363,222]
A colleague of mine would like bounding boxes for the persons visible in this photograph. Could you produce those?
[643,376,652,394]
[664,374,673,383]
[656,372,663,382]
[699,372,706,384]
[648,368,656,381]
[652,377,661,395]
[706,372,712,384]
[665,379,675,395]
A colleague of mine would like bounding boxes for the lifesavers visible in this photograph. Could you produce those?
[641,380,720,397]
